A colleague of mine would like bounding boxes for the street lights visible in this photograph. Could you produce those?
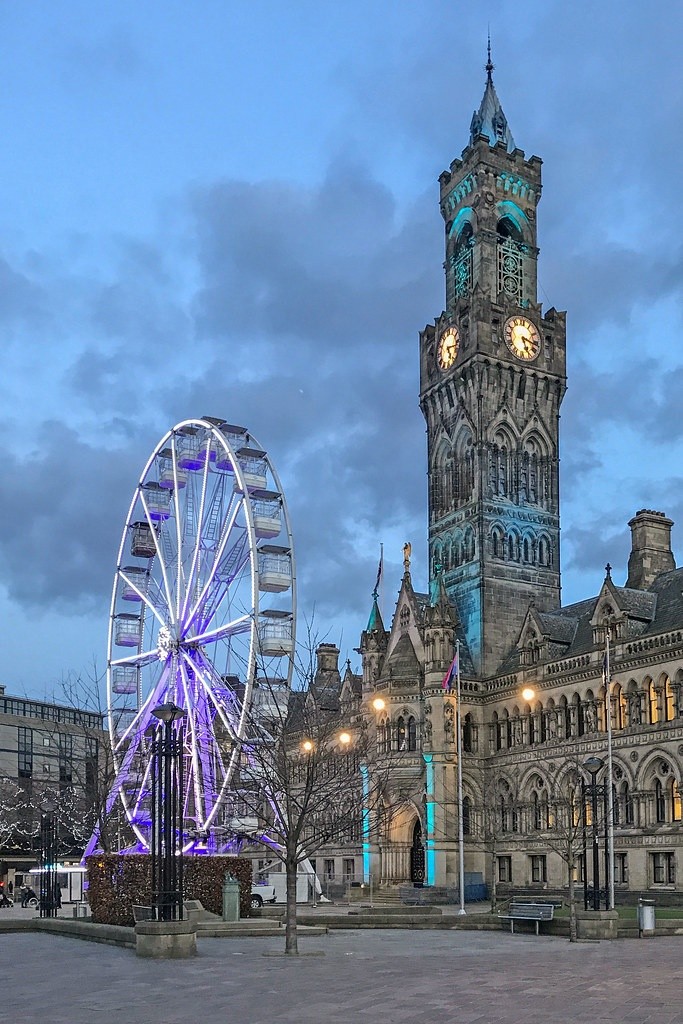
[585,756,604,912]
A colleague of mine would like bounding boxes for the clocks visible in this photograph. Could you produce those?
[435,326,460,371]
[503,315,542,362]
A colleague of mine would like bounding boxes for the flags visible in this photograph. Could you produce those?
[442,652,458,691]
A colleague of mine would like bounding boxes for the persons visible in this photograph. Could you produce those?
[0,880,13,908]
[54,882,63,909]
[20,883,36,908]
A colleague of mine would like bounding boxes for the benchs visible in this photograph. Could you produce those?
[400,888,426,906]
[498,903,554,937]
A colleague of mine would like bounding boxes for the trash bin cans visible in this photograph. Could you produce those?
[637,898,656,930]
